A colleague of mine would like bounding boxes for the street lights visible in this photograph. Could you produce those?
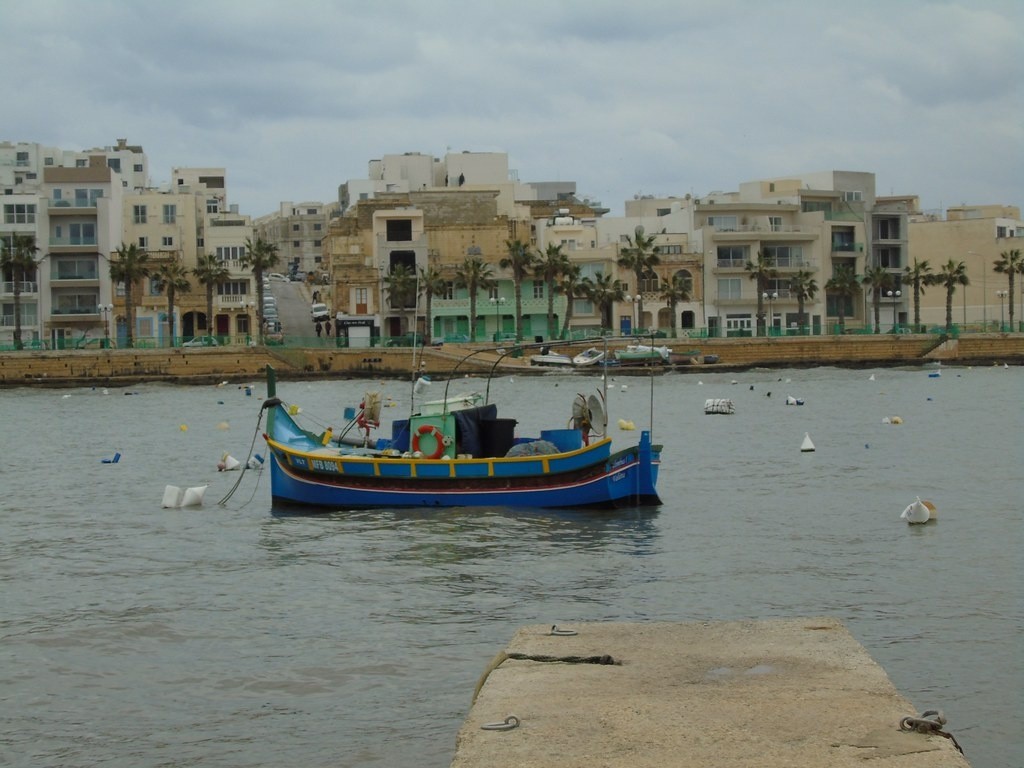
[239,301,254,346]
[763,292,778,336]
[97,304,113,348]
[626,295,641,334]
[489,297,505,342]
[997,291,1008,331]
[887,291,901,334]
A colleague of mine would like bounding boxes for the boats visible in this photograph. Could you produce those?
[529,346,701,367]
[262,363,665,509]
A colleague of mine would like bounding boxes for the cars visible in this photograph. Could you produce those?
[311,303,331,323]
[182,336,217,347]
[499,333,526,341]
[624,329,666,338]
[11,341,46,350]
[887,328,912,334]
[84,338,116,349]
[387,331,427,347]
[262,272,290,345]
[432,333,471,346]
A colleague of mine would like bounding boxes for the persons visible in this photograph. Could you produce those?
[312,290,322,304]
[262,317,268,347]
[325,320,331,336]
[573,393,592,447]
[316,320,322,338]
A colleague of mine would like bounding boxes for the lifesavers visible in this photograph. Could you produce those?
[411,424,446,460]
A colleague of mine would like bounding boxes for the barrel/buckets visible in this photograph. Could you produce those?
[512,438,540,445]
[376,438,392,450]
[477,419,519,457]
[541,429,583,454]
[392,420,410,452]
